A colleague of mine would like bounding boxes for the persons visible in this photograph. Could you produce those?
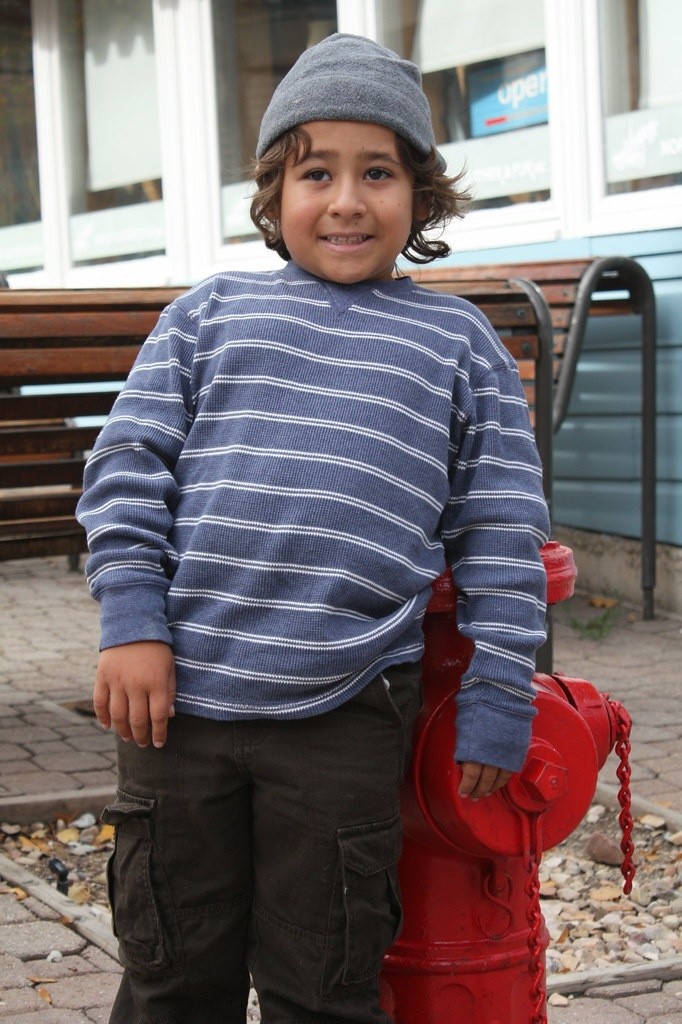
[94,34,547,1024]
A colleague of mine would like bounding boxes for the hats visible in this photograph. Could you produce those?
[254,32,446,173]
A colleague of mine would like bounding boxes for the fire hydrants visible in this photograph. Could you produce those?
[378,541,636,1024]
